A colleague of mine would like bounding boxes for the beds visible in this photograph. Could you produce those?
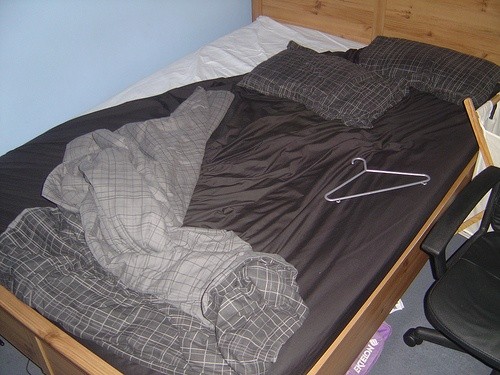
[2,2,499,374]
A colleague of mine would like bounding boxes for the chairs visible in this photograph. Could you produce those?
[403,167,500,374]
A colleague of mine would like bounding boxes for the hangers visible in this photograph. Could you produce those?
[324,156,434,205]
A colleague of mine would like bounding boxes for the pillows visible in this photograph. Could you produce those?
[233,40,410,133]
[355,32,500,106]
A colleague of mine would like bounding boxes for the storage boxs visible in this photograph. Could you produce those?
[454,93,500,239]
